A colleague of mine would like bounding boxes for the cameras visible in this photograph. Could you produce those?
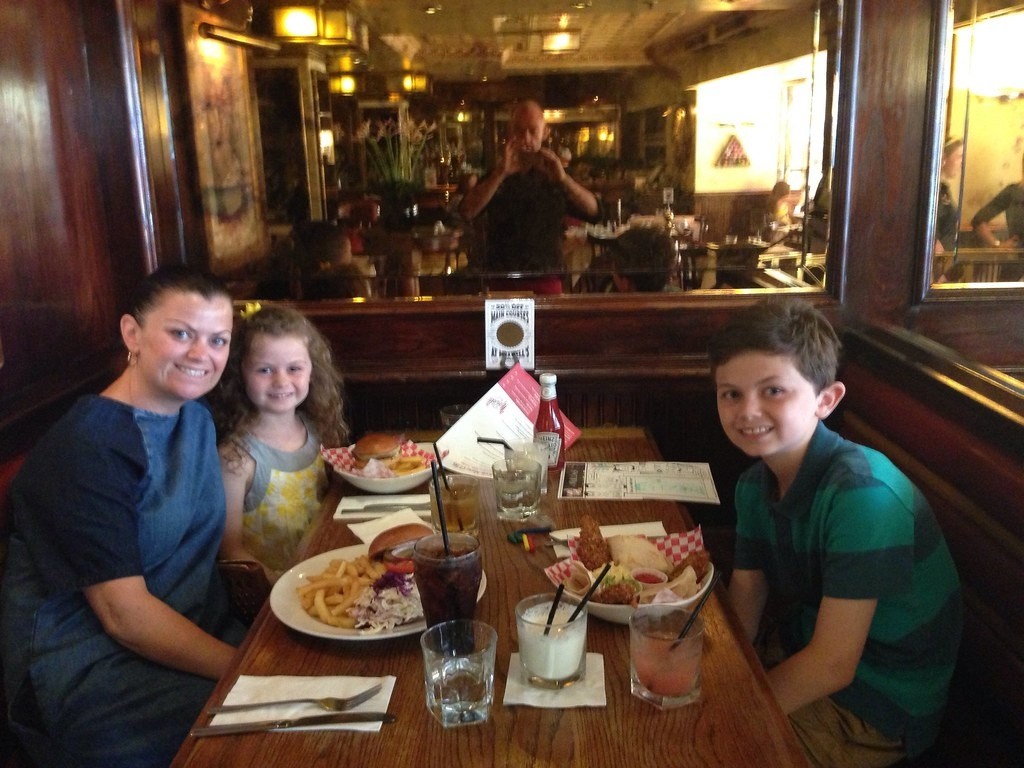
[519,150,544,166]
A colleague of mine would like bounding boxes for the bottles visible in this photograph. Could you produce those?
[533,373,566,473]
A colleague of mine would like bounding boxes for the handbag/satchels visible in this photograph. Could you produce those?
[215,560,269,628]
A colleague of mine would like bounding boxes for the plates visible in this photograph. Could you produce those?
[442,445,506,479]
[269,543,488,639]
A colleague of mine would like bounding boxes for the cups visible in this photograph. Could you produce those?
[412,532,483,656]
[428,473,481,538]
[627,603,705,711]
[515,592,589,691]
[726,235,739,245]
[594,220,617,236]
[491,457,542,522]
[419,618,497,728]
[504,439,550,496]
[438,403,472,432]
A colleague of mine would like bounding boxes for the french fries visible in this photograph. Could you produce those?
[392,454,426,476]
[295,556,389,626]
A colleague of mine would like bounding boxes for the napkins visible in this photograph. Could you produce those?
[211,674,397,732]
[347,507,436,544]
[549,520,668,559]
[503,650,607,710]
[332,493,432,519]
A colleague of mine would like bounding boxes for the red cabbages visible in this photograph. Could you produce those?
[374,569,414,598]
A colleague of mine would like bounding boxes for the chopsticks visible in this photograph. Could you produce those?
[542,563,610,635]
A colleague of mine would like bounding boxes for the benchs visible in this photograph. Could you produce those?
[0,345,162,576]
[830,328,1024,768]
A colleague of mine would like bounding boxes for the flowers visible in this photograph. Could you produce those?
[351,99,437,180]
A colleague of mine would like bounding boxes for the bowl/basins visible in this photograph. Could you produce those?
[631,566,667,591]
[542,555,714,625]
[334,442,442,492]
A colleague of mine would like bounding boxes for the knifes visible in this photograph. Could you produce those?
[189,712,398,737]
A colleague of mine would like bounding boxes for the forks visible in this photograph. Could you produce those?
[207,683,383,715]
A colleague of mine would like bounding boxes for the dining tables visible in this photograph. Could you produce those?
[170,425,820,768]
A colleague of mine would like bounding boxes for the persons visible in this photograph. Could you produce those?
[697,295,969,767]
[0,268,250,768]
[313,98,1024,296]
[204,303,351,591]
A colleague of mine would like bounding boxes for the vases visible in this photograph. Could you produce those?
[377,177,420,233]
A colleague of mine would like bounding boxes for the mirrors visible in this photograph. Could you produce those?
[82,0,863,316]
[914,0,1024,304]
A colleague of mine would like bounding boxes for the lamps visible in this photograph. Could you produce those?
[259,0,583,98]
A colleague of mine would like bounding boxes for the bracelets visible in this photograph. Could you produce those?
[992,237,999,247]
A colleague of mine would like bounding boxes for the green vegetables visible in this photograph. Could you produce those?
[593,561,642,592]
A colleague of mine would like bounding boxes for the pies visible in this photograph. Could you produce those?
[665,565,697,599]
[563,561,601,599]
[605,534,669,573]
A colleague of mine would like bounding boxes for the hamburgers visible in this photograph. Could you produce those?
[351,433,400,470]
[368,523,434,576]
